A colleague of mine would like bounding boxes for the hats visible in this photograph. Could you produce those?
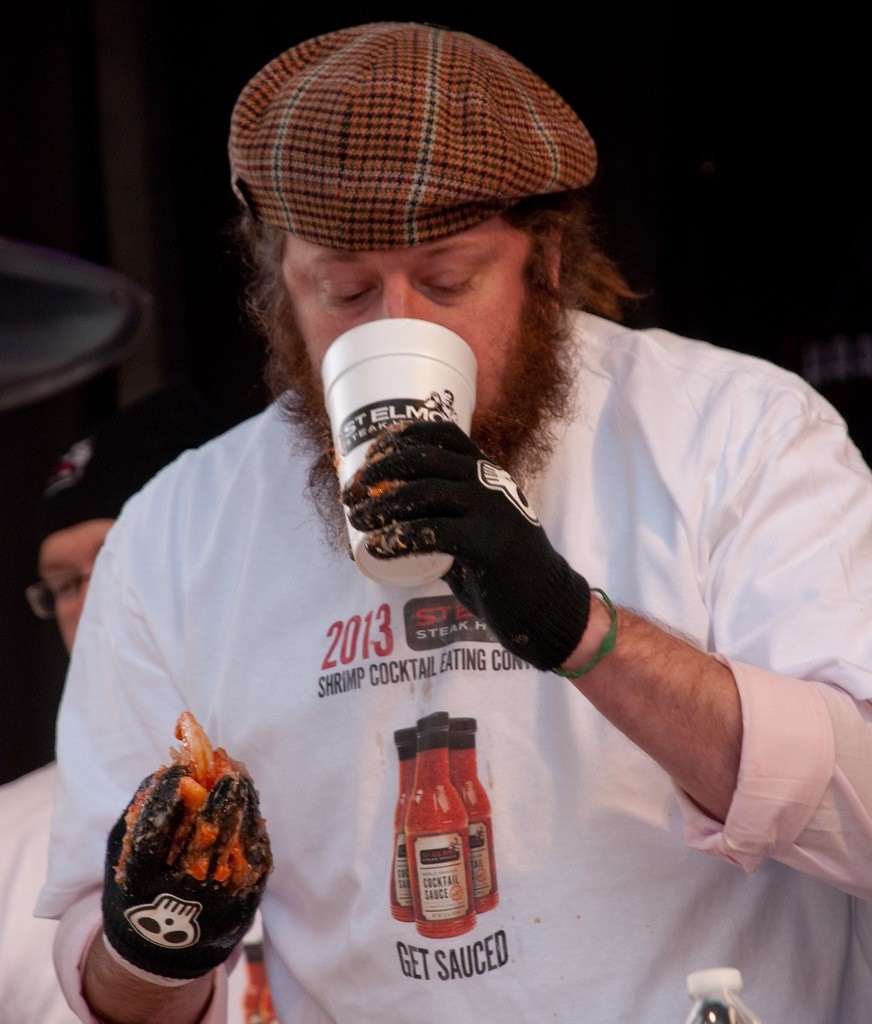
[32,424,191,547]
[229,22,599,252]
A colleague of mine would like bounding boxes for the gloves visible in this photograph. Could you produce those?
[342,421,590,671]
[100,764,275,988]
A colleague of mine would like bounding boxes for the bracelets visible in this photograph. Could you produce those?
[546,586,619,680]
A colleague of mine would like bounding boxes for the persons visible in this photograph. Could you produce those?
[31,9,871,1024]
[28,402,192,665]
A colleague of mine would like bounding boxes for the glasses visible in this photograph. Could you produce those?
[24,564,92,619]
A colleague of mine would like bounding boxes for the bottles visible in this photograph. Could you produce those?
[447,718,499,912]
[389,725,419,922]
[685,966,763,1024]
[405,711,476,939]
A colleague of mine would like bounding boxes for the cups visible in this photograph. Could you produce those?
[321,317,478,586]
